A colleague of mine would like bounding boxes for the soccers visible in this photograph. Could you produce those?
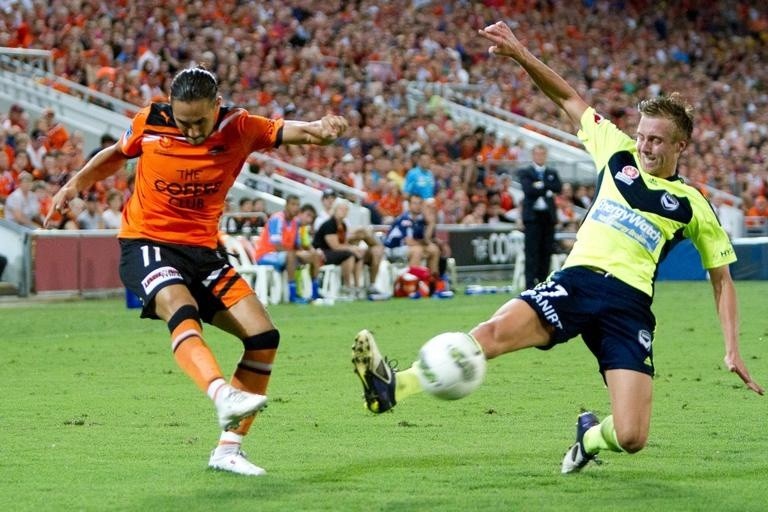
[420,331,487,401]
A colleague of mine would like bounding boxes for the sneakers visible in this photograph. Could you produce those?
[351,329,398,415]
[561,410,602,474]
[367,289,383,296]
[289,294,308,304]
[208,447,266,477]
[218,386,268,431]
[311,293,325,301]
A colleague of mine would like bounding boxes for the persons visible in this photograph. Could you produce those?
[43,64,349,477]
[352,19,764,474]
[0,0,768,305]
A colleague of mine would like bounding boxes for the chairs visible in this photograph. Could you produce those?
[514,228,568,295]
[222,231,458,306]
